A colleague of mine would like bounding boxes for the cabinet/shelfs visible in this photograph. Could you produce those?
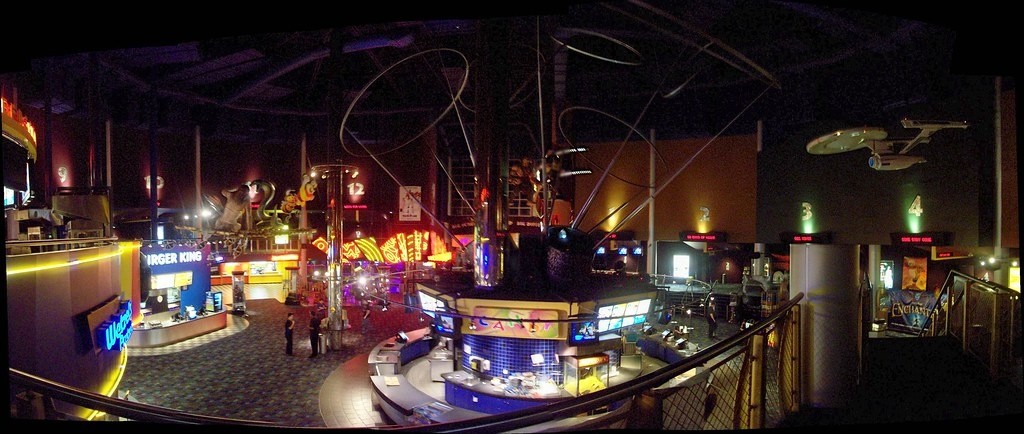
[562,352,609,397]
[427,358,454,383]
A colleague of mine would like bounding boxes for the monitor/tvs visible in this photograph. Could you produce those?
[568,321,598,347]
[676,338,684,345]
[662,329,672,338]
[435,313,462,333]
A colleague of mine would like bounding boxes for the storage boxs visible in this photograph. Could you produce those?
[620,342,642,370]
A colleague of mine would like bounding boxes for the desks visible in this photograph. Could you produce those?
[65,228,104,249]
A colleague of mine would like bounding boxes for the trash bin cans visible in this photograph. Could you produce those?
[317,333,327,355]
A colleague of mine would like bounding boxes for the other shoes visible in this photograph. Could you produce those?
[309,356,318,358]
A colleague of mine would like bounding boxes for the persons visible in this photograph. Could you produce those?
[284,313,295,355]
[706,302,718,338]
[306,311,321,359]
[674,326,682,340]
